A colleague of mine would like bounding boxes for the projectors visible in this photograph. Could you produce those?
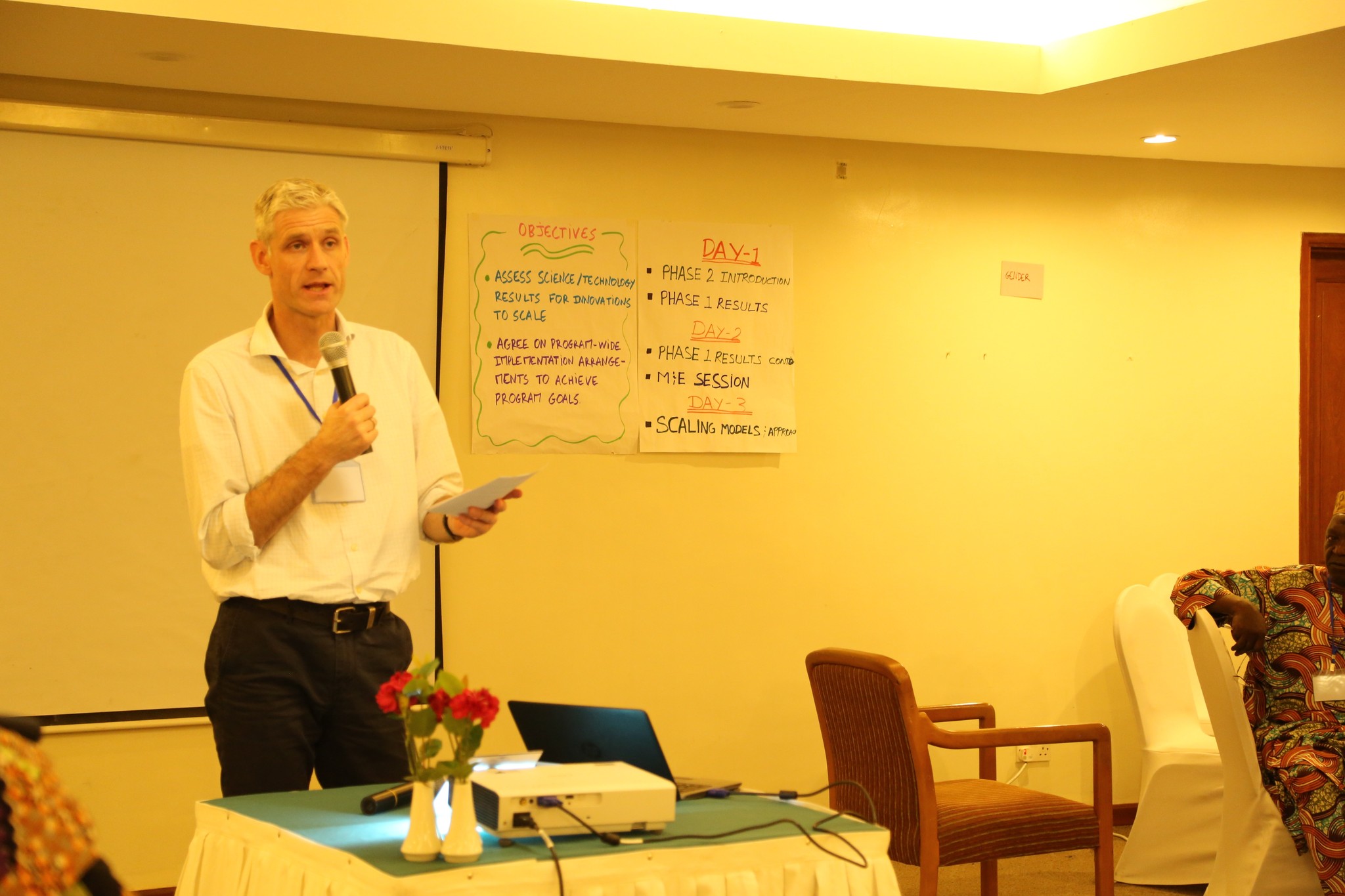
[448,758,677,840]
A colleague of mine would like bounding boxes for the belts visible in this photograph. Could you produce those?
[229,593,392,636]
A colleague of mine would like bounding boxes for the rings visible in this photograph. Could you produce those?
[369,418,376,429]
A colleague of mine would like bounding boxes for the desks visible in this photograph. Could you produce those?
[180,772,907,896]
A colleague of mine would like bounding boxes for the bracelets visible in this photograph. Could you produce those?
[442,513,463,541]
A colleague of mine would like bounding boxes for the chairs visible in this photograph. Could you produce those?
[1172,563,1345,894]
[805,644,1117,896]
[1110,571,1224,887]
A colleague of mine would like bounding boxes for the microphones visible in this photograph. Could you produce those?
[360,773,445,815]
[319,331,373,455]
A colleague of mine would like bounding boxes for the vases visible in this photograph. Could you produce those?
[378,664,496,865]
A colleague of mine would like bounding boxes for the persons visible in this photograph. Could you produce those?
[176,179,528,795]
[1172,488,1345,896]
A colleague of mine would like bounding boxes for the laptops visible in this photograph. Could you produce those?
[508,700,743,800]
